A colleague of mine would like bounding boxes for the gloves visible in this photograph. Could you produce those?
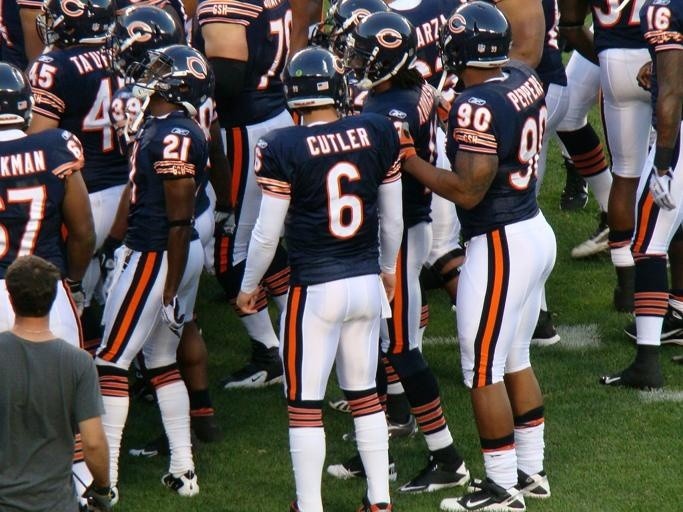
[66,279,85,318]
[394,120,417,163]
[94,234,122,304]
[161,294,186,336]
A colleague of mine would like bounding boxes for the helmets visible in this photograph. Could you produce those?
[0,1,213,130]
[283,0,512,109]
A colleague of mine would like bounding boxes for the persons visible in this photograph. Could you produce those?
[0,0,683,510]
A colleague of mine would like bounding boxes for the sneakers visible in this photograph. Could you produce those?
[561,150,682,389]
